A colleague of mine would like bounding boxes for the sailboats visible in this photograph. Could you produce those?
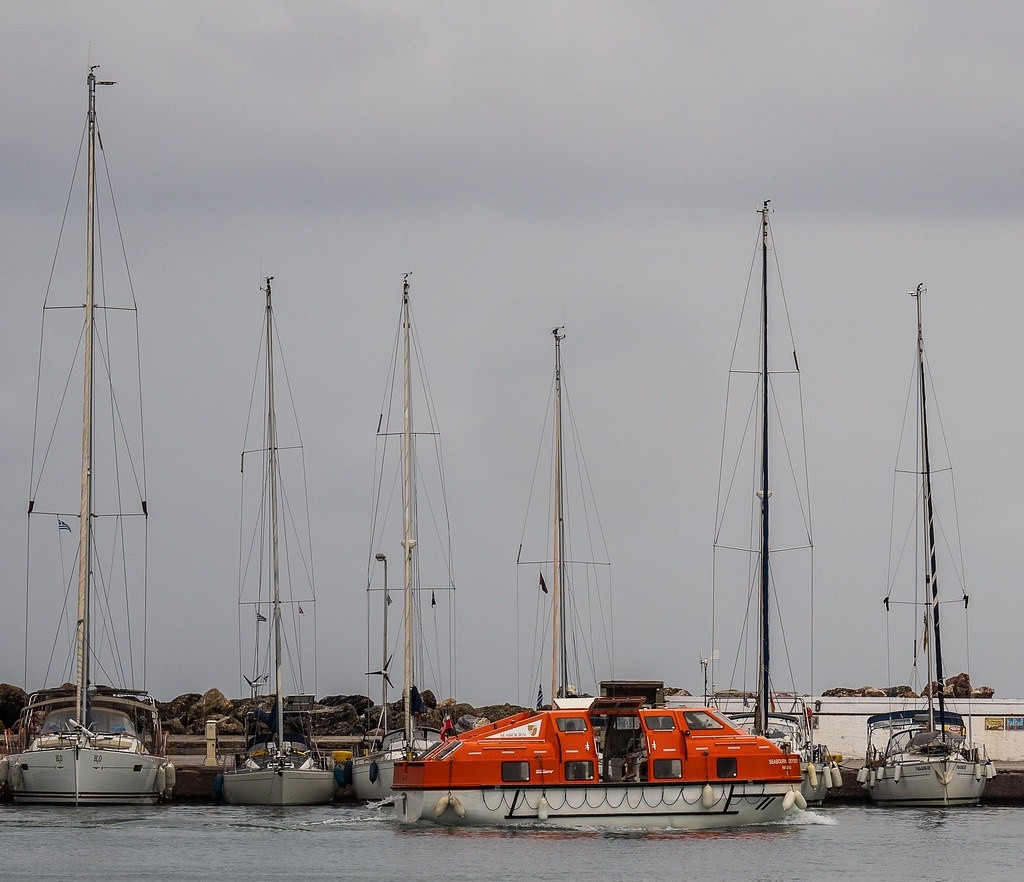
[8,65,167,804]
[352,271,630,807]
[710,199,830,809]
[866,284,986,804]
[219,274,339,806]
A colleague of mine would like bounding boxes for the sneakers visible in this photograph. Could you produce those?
[620,773,636,781]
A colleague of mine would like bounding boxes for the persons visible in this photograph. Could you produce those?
[620,723,644,781]
[566,722,602,779]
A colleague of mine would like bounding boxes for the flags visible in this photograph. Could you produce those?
[299,607,304,614]
[386,596,392,605]
[430,592,436,608]
[256,612,267,622]
[540,573,548,594]
[440,715,453,742]
[536,685,543,707]
[58,520,71,532]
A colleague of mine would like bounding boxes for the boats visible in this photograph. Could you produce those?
[395,699,802,834]
